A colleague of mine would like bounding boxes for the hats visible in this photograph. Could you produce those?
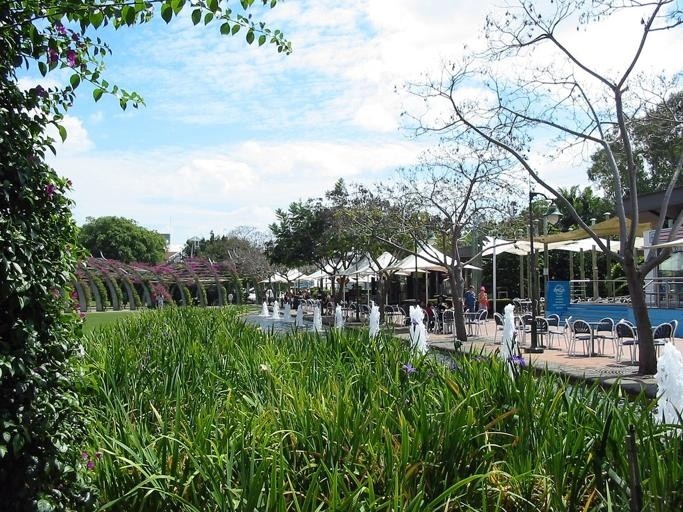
[480,286,485,291]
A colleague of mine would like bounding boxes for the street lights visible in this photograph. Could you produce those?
[415,225,437,306]
[525,190,563,353]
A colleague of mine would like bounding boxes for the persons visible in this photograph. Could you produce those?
[150,290,163,309]
[416,295,450,332]
[228,291,234,305]
[464,285,490,323]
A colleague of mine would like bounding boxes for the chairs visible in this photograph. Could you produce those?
[493,296,678,366]
[299,299,488,338]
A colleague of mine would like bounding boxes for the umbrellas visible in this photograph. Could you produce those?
[258,244,484,313]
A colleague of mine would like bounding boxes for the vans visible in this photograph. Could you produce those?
[242,286,275,304]
